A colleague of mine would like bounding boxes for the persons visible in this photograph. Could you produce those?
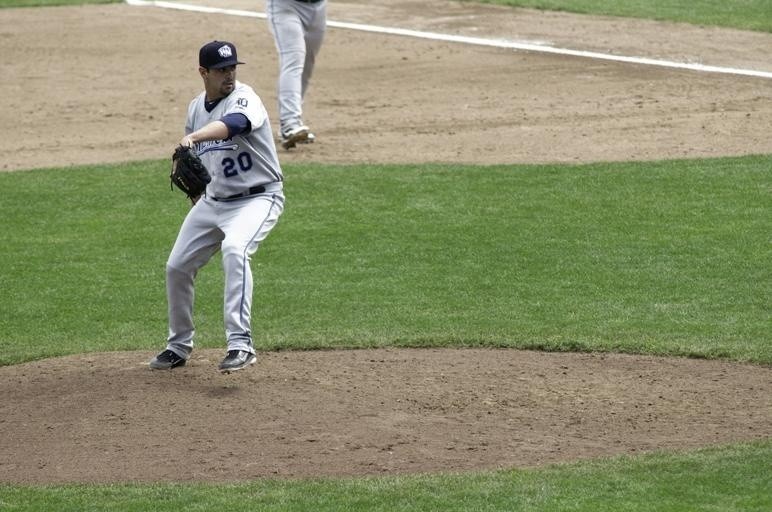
[148,40,287,372]
[264,1,328,151]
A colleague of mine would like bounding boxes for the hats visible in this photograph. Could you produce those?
[198,40,245,69]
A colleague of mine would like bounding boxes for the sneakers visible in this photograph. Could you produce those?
[218,350,256,372]
[149,350,186,371]
[281,126,315,150]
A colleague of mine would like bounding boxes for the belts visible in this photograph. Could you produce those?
[209,186,266,202]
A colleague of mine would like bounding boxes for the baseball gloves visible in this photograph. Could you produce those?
[170,143,212,200]
